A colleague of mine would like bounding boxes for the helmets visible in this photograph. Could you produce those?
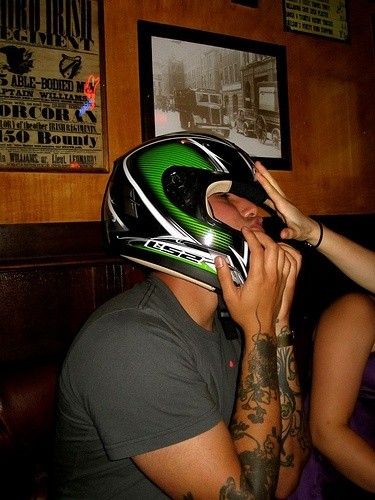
[102,130,269,296]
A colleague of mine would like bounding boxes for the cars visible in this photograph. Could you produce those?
[234,108,257,137]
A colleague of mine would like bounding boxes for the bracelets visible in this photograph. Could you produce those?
[306,218,323,249]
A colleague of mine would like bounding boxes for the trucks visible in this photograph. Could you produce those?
[174,88,232,137]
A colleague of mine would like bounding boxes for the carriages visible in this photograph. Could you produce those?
[256,81,281,150]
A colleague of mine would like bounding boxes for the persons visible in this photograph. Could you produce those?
[53,131,306,500]
[295,292,375,500]
[254,157,375,297]
[156,95,170,112]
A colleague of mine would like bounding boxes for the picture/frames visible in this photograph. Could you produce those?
[134,18,294,174]
[0,0,114,174]
[281,0,353,42]
